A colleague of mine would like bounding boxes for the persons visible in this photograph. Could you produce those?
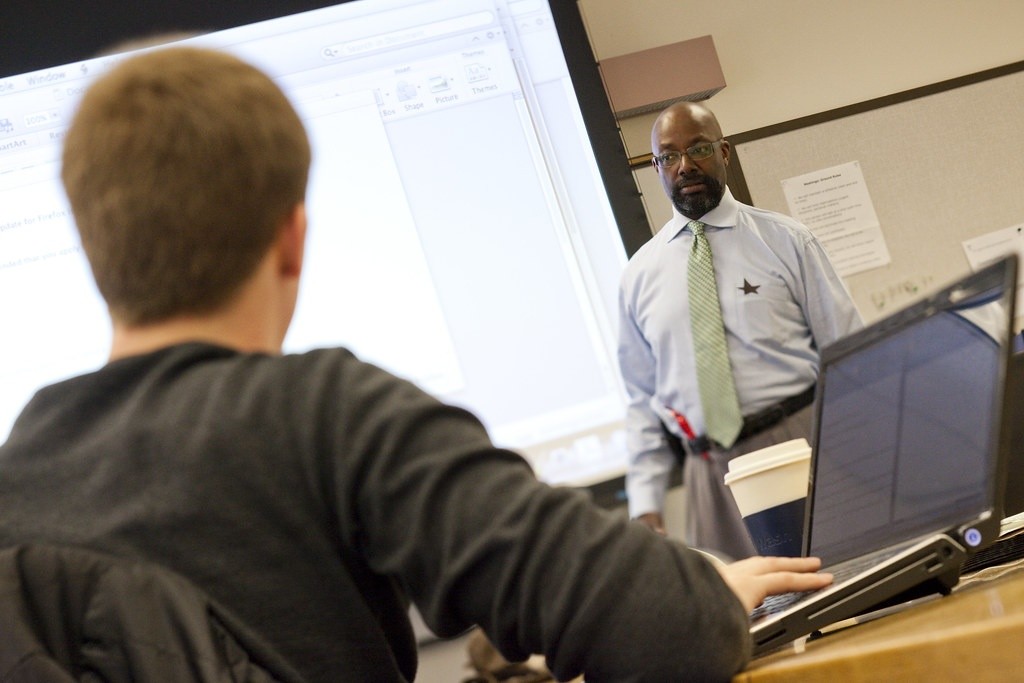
[618,102,865,561]
[0,49,833,683]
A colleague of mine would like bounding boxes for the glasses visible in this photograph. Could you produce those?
[652,137,724,169]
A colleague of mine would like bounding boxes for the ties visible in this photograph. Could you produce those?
[684,219,749,448]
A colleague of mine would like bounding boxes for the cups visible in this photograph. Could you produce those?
[723,437,812,559]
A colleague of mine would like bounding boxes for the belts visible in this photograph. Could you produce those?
[673,384,816,459]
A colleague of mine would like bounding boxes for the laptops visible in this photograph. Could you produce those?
[743,253,1019,661]
